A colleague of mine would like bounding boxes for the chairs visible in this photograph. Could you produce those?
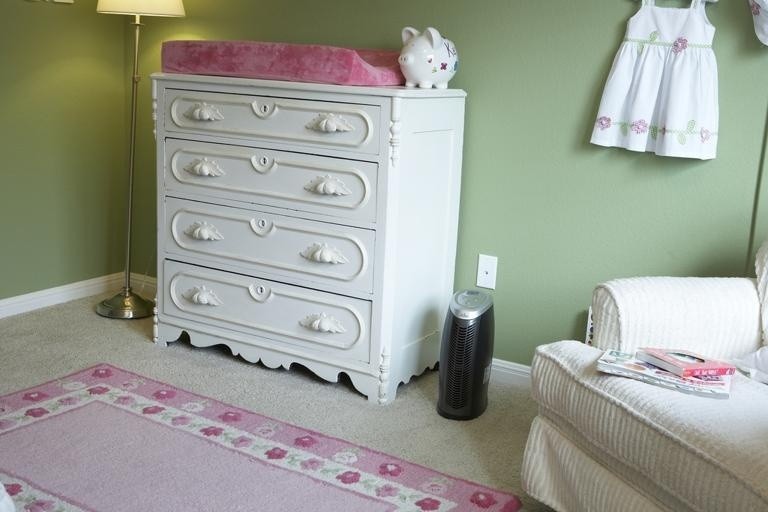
[521,242,768,512]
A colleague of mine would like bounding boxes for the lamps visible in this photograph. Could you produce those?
[95,0,186,319]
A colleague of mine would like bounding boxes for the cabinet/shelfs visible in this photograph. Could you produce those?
[149,71,466,406]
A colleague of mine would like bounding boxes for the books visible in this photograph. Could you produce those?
[634,344,737,379]
[596,349,732,398]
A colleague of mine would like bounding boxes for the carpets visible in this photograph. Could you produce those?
[0,363,522,512]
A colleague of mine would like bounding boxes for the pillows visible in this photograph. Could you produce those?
[726,346,768,385]
